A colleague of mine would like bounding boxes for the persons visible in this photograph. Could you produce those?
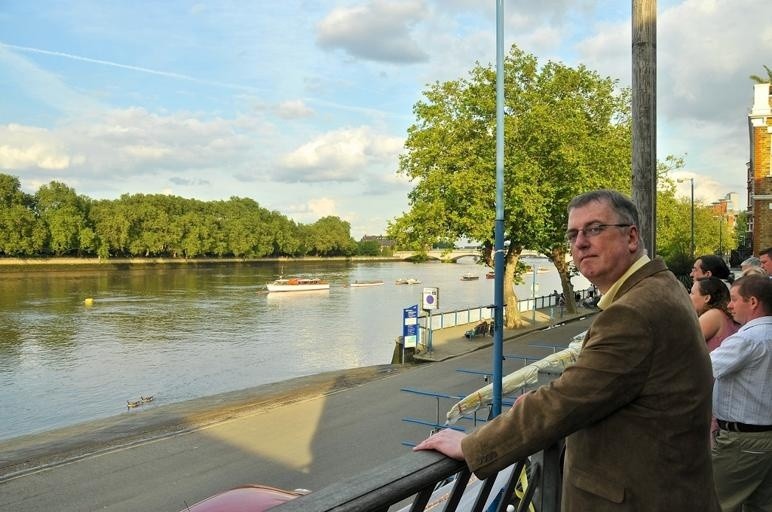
[547,282,598,309]
[689,247,772,512]
[413,191,724,511]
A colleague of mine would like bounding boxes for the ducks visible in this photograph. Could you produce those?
[126,399,140,408]
[140,395,154,403]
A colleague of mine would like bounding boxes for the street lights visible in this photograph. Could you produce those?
[676,176,693,264]
[713,215,722,258]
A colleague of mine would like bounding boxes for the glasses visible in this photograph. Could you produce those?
[563,224,631,244]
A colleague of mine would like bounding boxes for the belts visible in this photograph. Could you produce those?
[716,418,771,432]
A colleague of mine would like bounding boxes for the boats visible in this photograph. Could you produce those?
[460,265,551,281]
[266,278,331,291]
[350,277,422,287]
